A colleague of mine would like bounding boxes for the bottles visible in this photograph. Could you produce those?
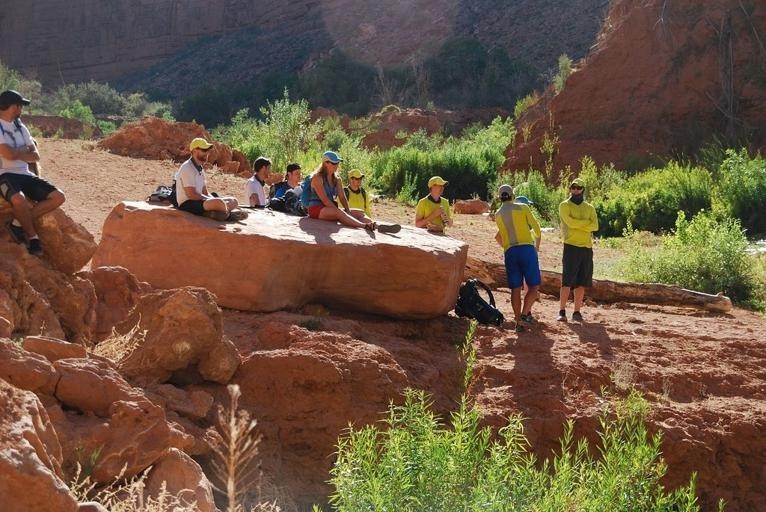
[440,206,449,225]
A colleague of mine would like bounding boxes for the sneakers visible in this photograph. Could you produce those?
[521,312,536,324]
[573,312,583,322]
[29,238,43,256]
[367,222,377,232]
[4,223,26,244]
[556,314,568,322]
[228,209,248,221]
[378,224,401,233]
[516,324,529,332]
[206,210,228,220]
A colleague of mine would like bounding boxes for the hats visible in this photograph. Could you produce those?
[349,169,365,179]
[0,89,30,106]
[499,184,515,198]
[516,196,533,204]
[285,163,301,180]
[322,151,343,163]
[189,138,214,152]
[571,178,586,188]
[428,176,449,188]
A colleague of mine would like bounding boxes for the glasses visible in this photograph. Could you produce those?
[331,162,339,165]
[572,186,582,190]
[355,178,362,180]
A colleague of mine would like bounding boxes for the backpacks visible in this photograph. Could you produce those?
[455,278,506,327]
[298,174,311,206]
[146,185,172,202]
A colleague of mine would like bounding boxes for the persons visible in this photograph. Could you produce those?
[494,196,534,322]
[246,151,402,233]
[175,138,248,221]
[0,89,65,256]
[556,178,599,321]
[415,176,453,233]
[494,184,541,332]
